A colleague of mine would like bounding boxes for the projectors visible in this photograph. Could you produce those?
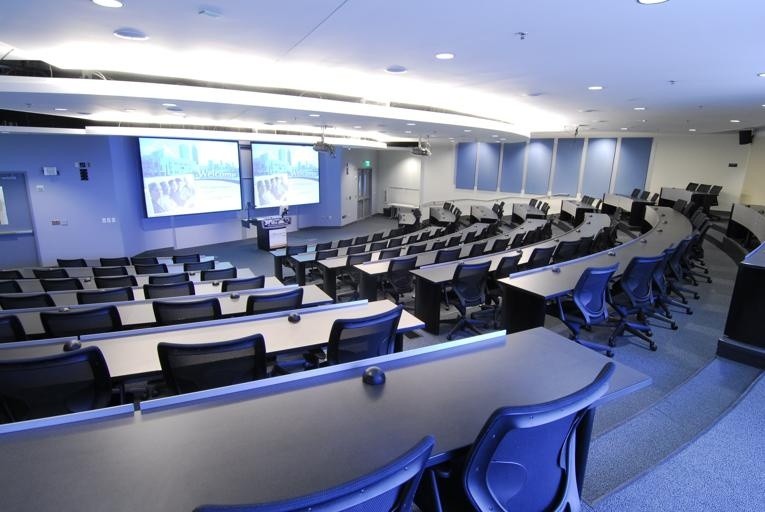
[410,148,432,158]
[313,143,330,152]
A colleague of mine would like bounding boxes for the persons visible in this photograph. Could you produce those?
[257,175,288,205]
[148,177,194,213]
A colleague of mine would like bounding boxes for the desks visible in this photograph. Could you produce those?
[716,202,765,371]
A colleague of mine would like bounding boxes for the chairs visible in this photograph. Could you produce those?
[0,182,724,512]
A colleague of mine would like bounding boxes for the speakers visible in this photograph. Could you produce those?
[739,130,752,144]
